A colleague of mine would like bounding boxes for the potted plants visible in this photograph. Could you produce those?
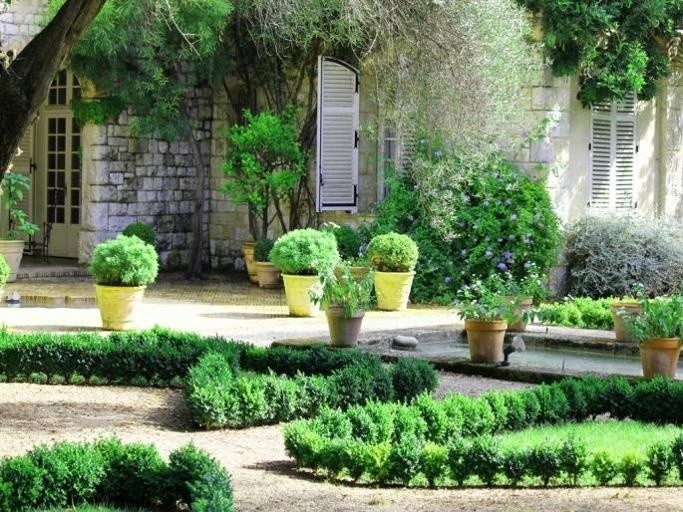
[219,103,315,289]
[617,294,682,384]
[268,225,418,317]
[0,148,40,281]
[88,233,160,332]
[309,261,379,346]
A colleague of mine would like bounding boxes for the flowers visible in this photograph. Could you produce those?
[456,260,547,323]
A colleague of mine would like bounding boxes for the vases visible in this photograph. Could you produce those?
[462,317,506,364]
[497,297,532,330]
[613,301,644,341]
